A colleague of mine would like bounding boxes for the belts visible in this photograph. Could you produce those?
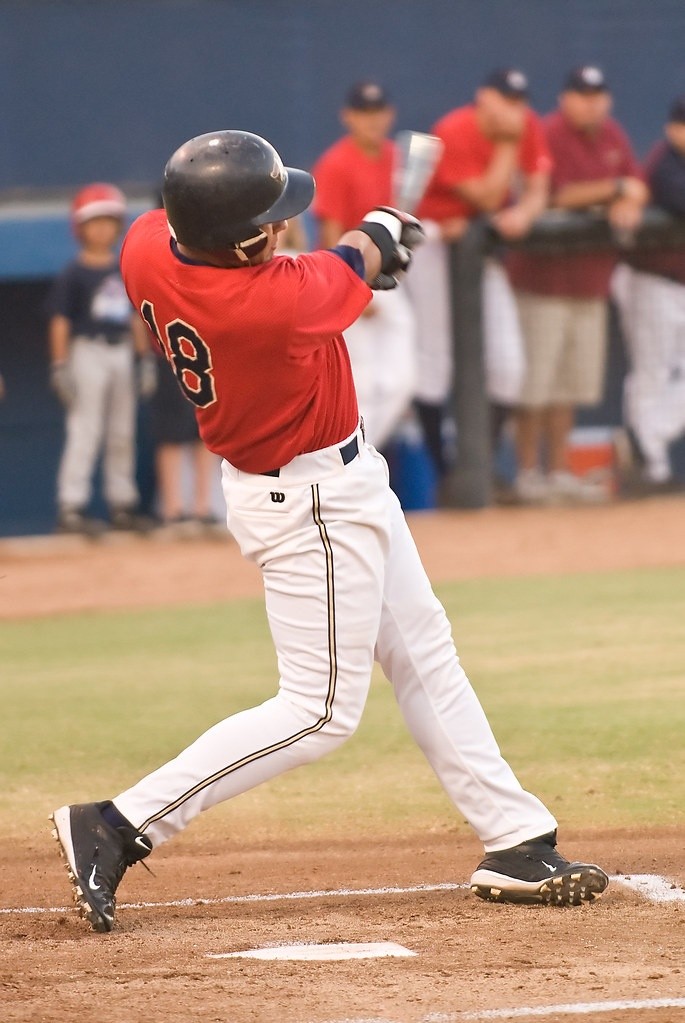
[257,417,366,479]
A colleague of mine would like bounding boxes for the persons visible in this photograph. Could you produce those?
[46,129,611,934]
[151,355,224,529]
[47,182,156,533]
[275,67,684,508]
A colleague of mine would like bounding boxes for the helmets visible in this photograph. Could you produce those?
[72,180,126,226]
[163,130,316,260]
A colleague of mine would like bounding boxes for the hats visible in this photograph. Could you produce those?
[484,67,530,97]
[348,83,388,111]
[569,67,606,93]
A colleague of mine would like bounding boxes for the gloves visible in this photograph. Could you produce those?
[360,204,425,290]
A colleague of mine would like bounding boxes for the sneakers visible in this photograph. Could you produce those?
[49,800,152,932]
[469,829,609,907]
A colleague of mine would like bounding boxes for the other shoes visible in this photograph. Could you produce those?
[517,471,548,499]
[555,472,605,499]
[56,509,98,534]
[116,511,159,530]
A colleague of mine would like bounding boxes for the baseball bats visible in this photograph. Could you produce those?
[374,130,447,291]
